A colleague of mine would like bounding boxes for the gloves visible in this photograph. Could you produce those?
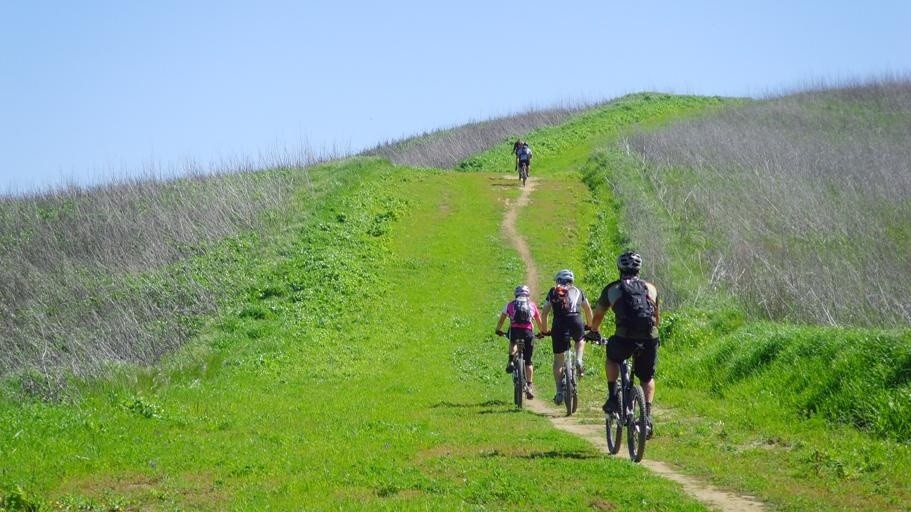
[584,331,599,343]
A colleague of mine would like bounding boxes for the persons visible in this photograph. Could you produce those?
[511,137,527,170]
[517,143,533,181]
[583,251,660,442]
[541,269,593,406]
[494,284,542,400]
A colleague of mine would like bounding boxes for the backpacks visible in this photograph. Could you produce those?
[553,284,571,313]
[513,298,532,324]
[620,276,654,328]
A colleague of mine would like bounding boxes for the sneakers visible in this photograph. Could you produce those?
[505,359,621,412]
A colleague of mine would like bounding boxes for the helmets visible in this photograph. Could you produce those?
[516,285,529,297]
[555,270,573,282]
[618,252,642,273]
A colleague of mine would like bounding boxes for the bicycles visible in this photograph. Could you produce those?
[496,329,550,410]
[518,164,529,187]
[588,334,661,463]
[542,327,595,416]
